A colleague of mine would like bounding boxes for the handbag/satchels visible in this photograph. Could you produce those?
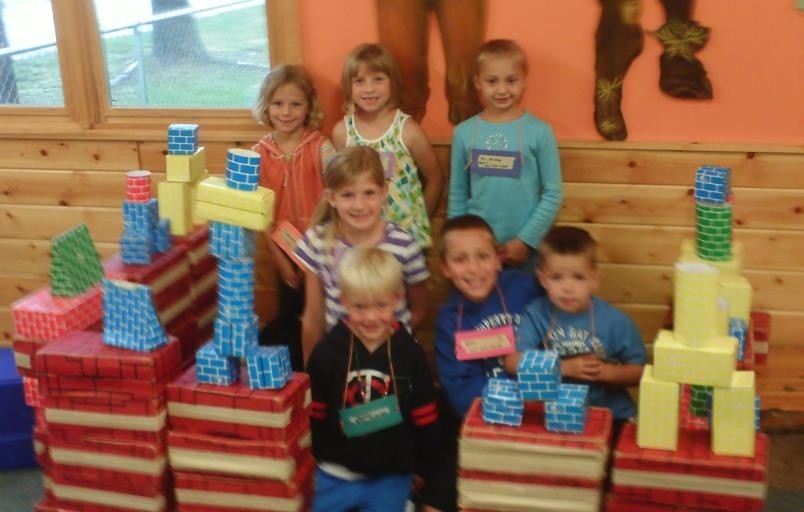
[455,326,516,362]
[340,395,405,439]
[271,221,310,271]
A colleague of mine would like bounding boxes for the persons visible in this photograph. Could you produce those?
[432,212,540,421]
[305,244,444,512]
[499,225,647,424]
[330,41,444,250]
[589,0,717,146]
[371,1,490,128]
[249,63,337,376]
[444,38,564,269]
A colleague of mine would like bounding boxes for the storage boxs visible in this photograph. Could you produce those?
[0,347,40,472]
